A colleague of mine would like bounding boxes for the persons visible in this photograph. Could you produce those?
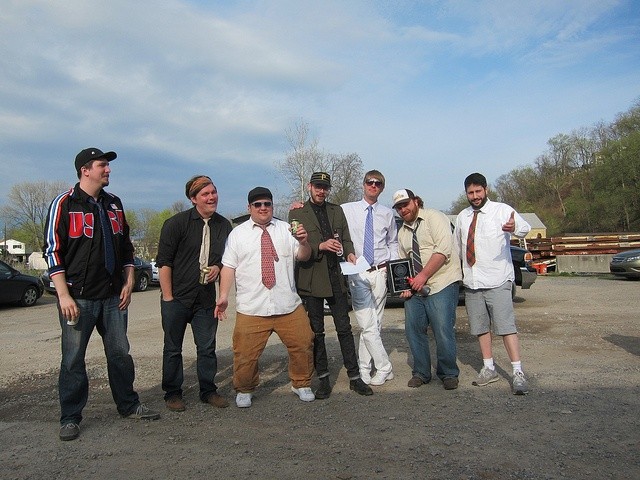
[289,169,400,386]
[288,171,374,400]
[41,147,161,440]
[391,188,463,391]
[452,172,532,396]
[213,186,316,408]
[154,175,232,412]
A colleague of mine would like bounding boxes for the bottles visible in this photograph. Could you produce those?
[333,228,345,262]
[405,276,431,296]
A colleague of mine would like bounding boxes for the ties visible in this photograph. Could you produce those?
[198,216,211,286]
[363,206,374,265]
[403,218,424,285]
[254,223,279,290]
[88,196,116,276]
[466,210,481,268]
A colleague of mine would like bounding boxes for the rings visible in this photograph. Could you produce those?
[333,242,335,245]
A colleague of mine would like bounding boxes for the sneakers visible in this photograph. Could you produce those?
[407,375,424,388]
[511,371,529,396]
[235,390,253,408]
[360,372,372,385]
[59,423,80,441]
[292,386,315,402]
[370,371,394,386]
[472,367,501,387]
[442,377,460,390]
[349,377,374,396]
[118,401,161,422]
[314,383,332,399]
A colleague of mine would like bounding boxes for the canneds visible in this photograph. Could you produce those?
[291,219,301,235]
[66,308,79,327]
[199,267,210,285]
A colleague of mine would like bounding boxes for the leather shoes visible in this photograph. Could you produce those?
[207,393,230,408]
[164,399,186,412]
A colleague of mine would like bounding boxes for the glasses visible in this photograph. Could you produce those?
[364,179,383,188]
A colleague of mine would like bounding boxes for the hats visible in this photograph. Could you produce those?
[74,148,117,170]
[248,186,273,201]
[391,188,416,209]
[310,171,333,187]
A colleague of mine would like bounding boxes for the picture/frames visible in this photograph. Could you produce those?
[386,258,415,297]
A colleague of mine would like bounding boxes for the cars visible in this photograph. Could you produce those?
[610,249,640,280]
[40,255,153,296]
[1,260,44,307]
[510,246,537,299]
